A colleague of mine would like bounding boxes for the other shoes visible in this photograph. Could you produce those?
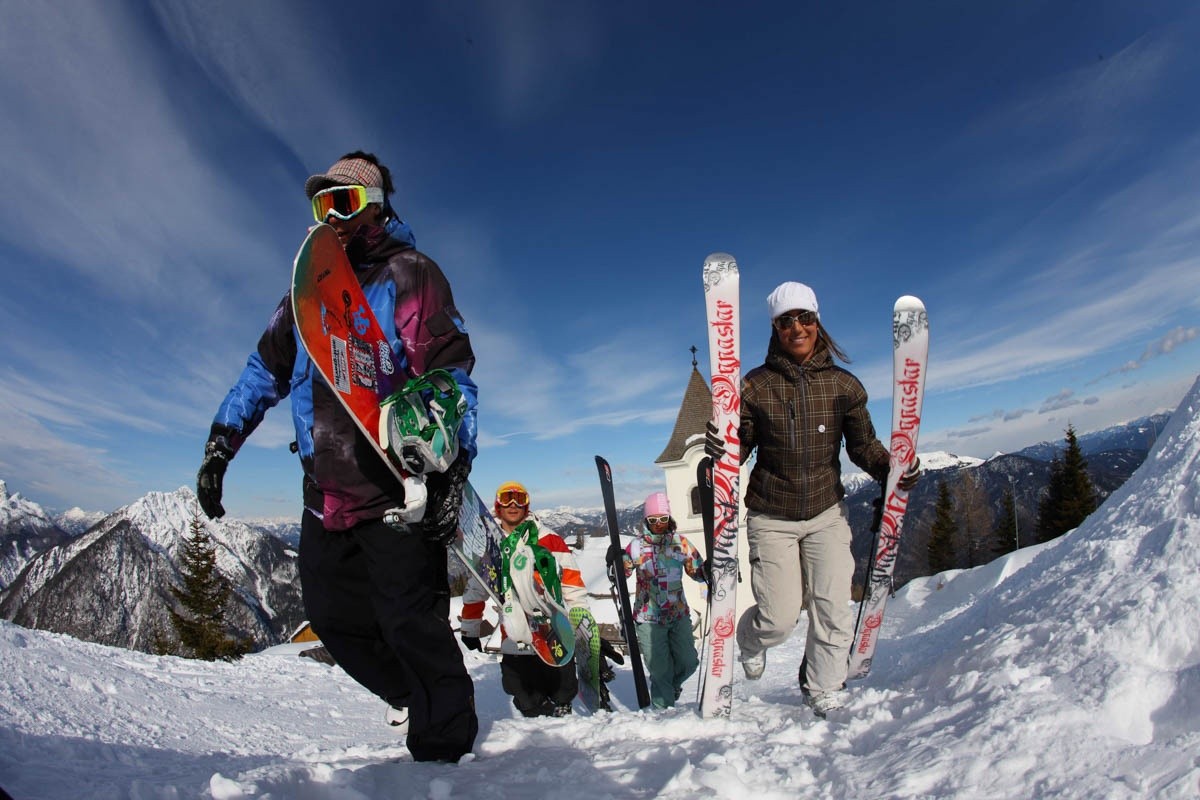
[553,704,572,717]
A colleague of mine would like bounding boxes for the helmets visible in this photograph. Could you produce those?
[495,482,529,518]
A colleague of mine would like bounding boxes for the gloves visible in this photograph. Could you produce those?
[896,456,920,491]
[606,544,625,567]
[461,636,482,652]
[420,470,459,547]
[704,418,726,460]
[195,451,227,520]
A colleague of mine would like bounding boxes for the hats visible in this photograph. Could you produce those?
[305,159,383,200]
[644,493,671,518]
[767,281,818,318]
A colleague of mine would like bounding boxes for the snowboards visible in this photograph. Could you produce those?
[567,606,624,715]
[289,224,576,667]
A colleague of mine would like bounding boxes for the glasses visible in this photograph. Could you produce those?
[312,185,384,224]
[775,311,815,329]
[495,489,529,507]
[646,515,669,524]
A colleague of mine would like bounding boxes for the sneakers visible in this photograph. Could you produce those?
[804,683,850,719]
[743,650,765,680]
[386,705,409,726]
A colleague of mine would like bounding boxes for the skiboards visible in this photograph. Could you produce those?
[592,454,713,709]
[695,250,930,721]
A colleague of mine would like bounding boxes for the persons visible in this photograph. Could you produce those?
[460,482,590,717]
[196,150,480,763]
[605,493,742,717]
[705,279,922,719]
[599,637,624,711]
